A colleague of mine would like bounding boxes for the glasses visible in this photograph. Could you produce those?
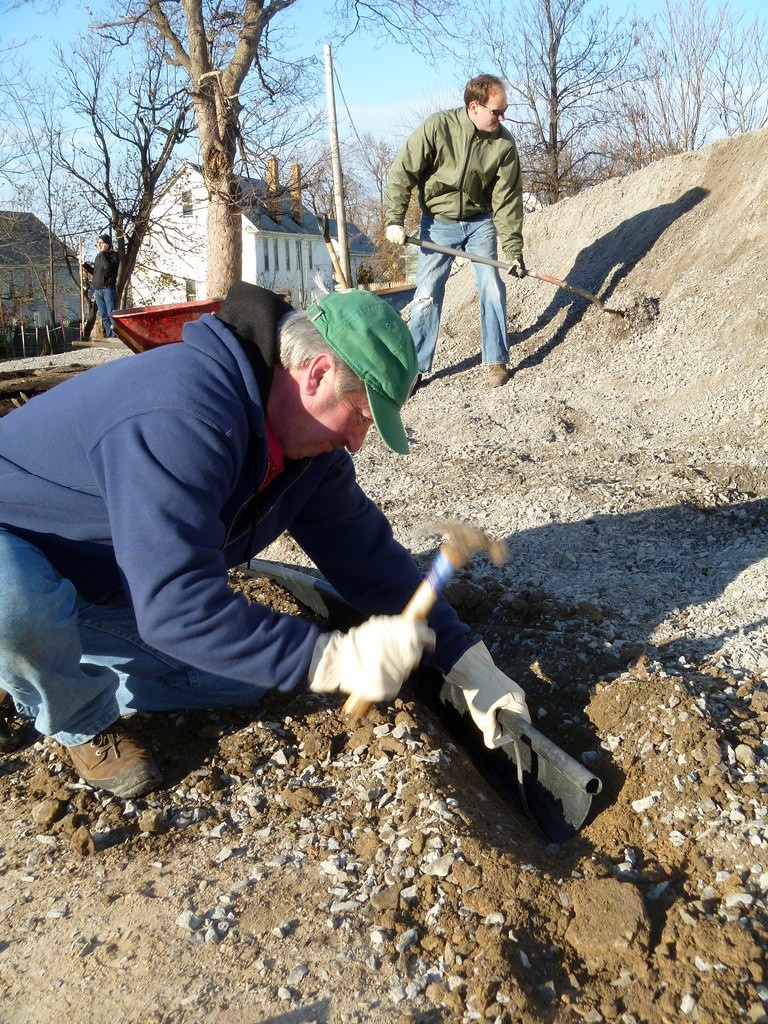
[474,99,508,117]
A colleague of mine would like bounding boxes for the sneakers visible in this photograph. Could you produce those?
[0,693,43,752]
[488,364,508,387]
[66,716,162,798]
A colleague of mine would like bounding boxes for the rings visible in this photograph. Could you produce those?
[105,244,108,246]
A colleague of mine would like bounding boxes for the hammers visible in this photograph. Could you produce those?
[341,522,509,719]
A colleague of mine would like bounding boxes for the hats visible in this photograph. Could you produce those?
[306,288,419,455]
[100,234,111,249]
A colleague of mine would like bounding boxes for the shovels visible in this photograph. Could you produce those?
[406,236,624,315]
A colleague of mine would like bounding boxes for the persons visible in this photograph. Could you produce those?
[385,73,528,401]
[0,282,533,802]
[79,234,121,338]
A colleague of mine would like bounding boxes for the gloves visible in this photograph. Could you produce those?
[386,224,406,247]
[506,258,525,279]
[442,639,531,749]
[307,615,436,701]
